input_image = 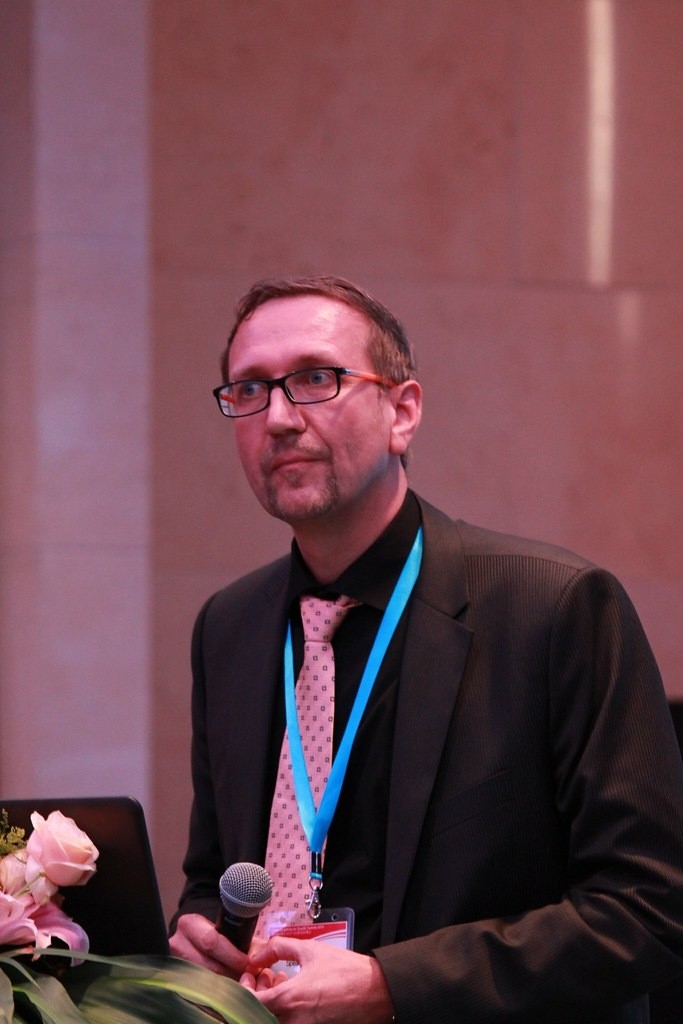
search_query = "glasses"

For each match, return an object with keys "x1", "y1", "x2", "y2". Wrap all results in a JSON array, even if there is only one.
[{"x1": 212, "y1": 365, "x2": 398, "y2": 418}]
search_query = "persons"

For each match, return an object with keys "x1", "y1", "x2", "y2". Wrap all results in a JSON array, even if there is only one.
[{"x1": 165, "y1": 275, "x2": 683, "y2": 1024}]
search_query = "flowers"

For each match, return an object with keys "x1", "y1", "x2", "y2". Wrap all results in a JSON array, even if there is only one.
[{"x1": 0, "y1": 807, "x2": 281, "y2": 1024}]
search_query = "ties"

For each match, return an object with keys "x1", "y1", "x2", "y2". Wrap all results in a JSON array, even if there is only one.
[{"x1": 252, "y1": 595, "x2": 363, "y2": 938}]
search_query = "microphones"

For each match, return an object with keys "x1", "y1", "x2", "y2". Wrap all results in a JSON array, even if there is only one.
[{"x1": 214, "y1": 862, "x2": 274, "y2": 955}]
